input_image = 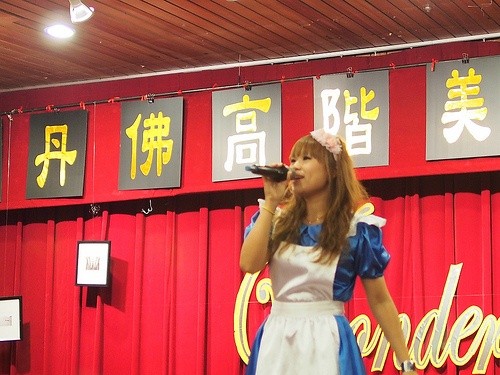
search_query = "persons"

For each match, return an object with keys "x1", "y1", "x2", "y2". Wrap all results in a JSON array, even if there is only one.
[{"x1": 238, "y1": 128, "x2": 416, "y2": 375}]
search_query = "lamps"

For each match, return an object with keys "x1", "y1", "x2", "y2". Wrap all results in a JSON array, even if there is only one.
[{"x1": 68, "y1": 0, "x2": 96, "y2": 25}]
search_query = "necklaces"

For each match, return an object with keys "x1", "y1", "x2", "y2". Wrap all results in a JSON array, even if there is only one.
[{"x1": 304, "y1": 212, "x2": 327, "y2": 225}]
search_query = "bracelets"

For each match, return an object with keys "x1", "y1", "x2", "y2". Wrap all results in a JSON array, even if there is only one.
[
  {"x1": 402, "y1": 360, "x2": 413, "y2": 372},
  {"x1": 263, "y1": 207, "x2": 275, "y2": 215}
]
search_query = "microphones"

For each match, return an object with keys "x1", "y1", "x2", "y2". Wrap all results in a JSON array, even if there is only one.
[{"x1": 245, "y1": 164, "x2": 296, "y2": 181}]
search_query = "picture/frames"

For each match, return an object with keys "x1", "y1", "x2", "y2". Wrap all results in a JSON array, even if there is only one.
[
  {"x1": 74, "y1": 240, "x2": 111, "y2": 286},
  {"x1": 0, "y1": 296, "x2": 23, "y2": 342}
]
